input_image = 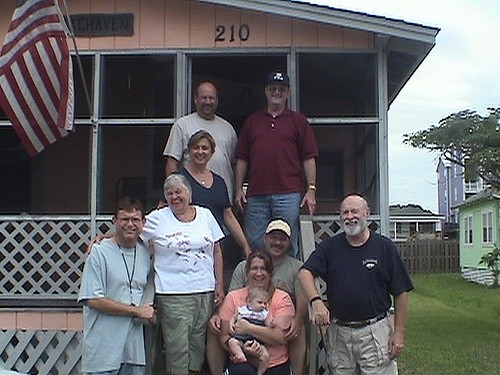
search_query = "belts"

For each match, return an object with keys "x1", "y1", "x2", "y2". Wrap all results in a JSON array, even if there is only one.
[{"x1": 329, "y1": 312, "x2": 388, "y2": 329}]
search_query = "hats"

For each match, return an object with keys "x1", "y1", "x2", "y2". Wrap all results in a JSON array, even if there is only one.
[
  {"x1": 265, "y1": 220, "x2": 292, "y2": 237},
  {"x1": 266, "y1": 70, "x2": 289, "y2": 86}
]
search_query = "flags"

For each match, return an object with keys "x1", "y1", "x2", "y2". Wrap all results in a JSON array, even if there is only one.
[{"x1": 0, "y1": 1, "x2": 74, "y2": 158}]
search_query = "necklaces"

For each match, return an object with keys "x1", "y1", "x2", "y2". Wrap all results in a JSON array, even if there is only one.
[
  {"x1": 190, "y1": 167, "x2": 208, "y2": 184},
  {"x1": 115, "y1": 234, "x2": 136, "y2": 307}
]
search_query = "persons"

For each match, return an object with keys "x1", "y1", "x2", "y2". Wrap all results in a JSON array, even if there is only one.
[
  {"x1": 87, "y1": 172, "x2": 227, "y2": 375},
  {"x1": 157, "y1": 130, "x2": 252, "y2": 258},
  {"x1": 218, "y1": 251, "x2": 295, "y2": 375},
  {"x1": 227, "y1": 288, "x2": 270, "y2": 375},
  {"x1": 78, "y1": 198, "x2": 155, "y2": 375},
  {"x1": 162, "y1": 82, "x2": 240, "y2": 257},
  {"x1": 207, "y1": 220, "x2": 307, "y2": 375},
  {"x1": 297, "y1": 195, "x2": 414, "y2": 375},
  {"x1": 235, "y1": 69, "x2": 319, "y2": 259}
]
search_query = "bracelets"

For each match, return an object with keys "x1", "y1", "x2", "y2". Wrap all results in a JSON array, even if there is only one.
[
  {"x1": 310, "y1": 297, "x2": 321, "y2": 307},
  {"x1": 307, "y1": 184, "x2": 316, "y2": 190}
]
